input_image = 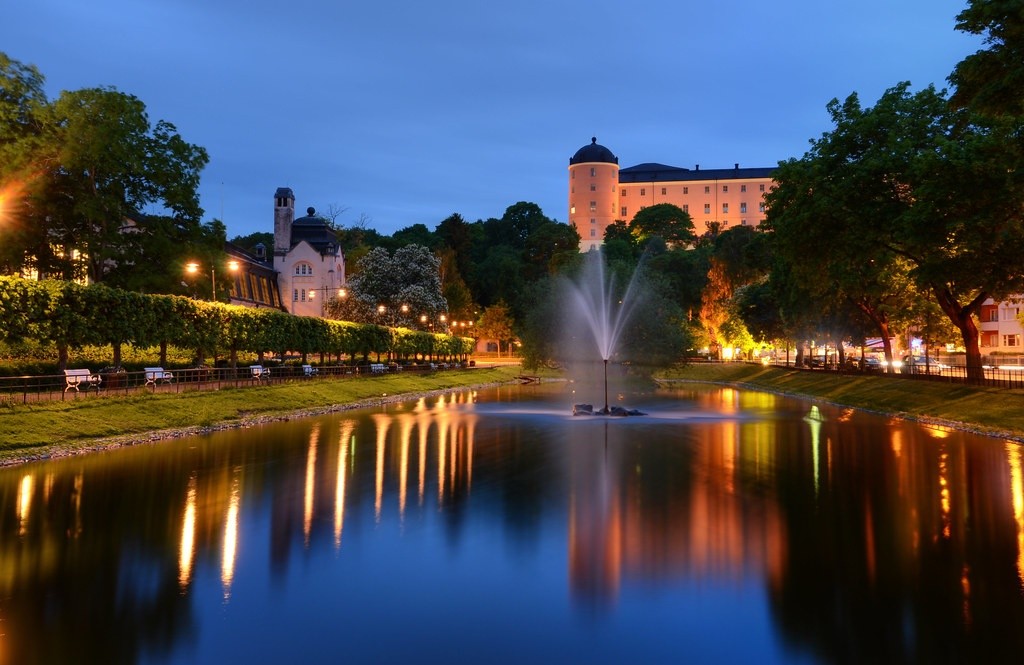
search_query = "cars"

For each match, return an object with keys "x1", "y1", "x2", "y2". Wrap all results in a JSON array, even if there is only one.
[
  {"x1": 852, "y1": 358, "x2": 860, "y2": 366},
  {"x1": 808, "y1": 355, "x2": 829, "y2": 367},
  {"x1": 864, "y1": 358, "x2": 881, "y2": 366}
]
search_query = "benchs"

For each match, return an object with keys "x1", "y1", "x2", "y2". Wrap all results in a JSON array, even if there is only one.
[
  {"x1": 249, "y1": 365, "x2": 270, "y2": 382},
  {"x1": 302, "y1": 365, "x2": 318, "y2": 377},
  {"x1": 369, "y1": 363, "x2": 462, "y2": 374},
  {"x1": 143, "y1": 367, "x2": 172, "y2": 386},
  {"x1": 63, "y1": 369, "x2": 102, "y2": 392}
]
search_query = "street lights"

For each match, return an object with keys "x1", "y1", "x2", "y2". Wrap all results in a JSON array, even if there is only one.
[
  {"x1": 185, "y1": 261, "x2": 240, "y2": 302},
  {"x1": 379, "y1": 305, "x2": 408, "y2": 328},
  {"x1": 421, "y1": 315, "x2": 445, "y2": 333},
  {"x1": 309, "y1": 288, "x2": 346, "y2": 320}
]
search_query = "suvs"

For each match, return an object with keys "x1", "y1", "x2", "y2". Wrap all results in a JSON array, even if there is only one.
[{"x1": 900, "y1": 355, "x2": 942, "y2": 375}]
